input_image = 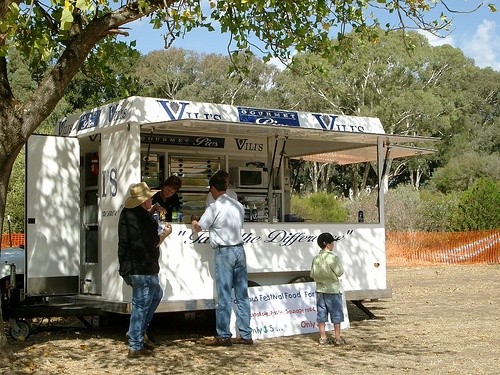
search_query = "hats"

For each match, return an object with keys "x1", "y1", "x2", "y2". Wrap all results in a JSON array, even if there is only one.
[
  {"x1": 318, "y1": 232, "x2": 340, "y2": 248},
  {"x1": 124, "y1": 182, "x2": 162, "y2": 208}
]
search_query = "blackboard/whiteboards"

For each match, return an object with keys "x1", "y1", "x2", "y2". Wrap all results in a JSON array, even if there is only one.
[{"x1": 229, "y1": 279, "x2": 351, "y2": 339}]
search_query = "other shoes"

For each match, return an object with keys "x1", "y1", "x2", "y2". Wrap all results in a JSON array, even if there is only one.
[
  {"x1": 129, "y1": 346, "x2": 150, "y2": 357},
  {"x1": 143, "y1": 344, "x2": 154, "y2": 350},
  {"x1": 336, "y1": 338, "x2": 346, "y2": 345},
  {"x1": 320, "y1": 338, "x2": 329, "y2": 345},
  {"x1": 234, "y1": 338, "x2": 252, "y2": 344},
  {"x1": 206, "y1": 337, "x2": 231, "y2": 346}
]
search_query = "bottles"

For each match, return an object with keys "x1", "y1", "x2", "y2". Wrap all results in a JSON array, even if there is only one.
[
  {"x1": 171, "y1": 207, "x2": 183, "y2": 223},
  {"x1": 243, "y1": 204, "x2": 258, "y2": 222},
  {"x1": 153, "y1": 210, "x2": 159, "y2": 222},
  {"x1": 358, "y1": 209, "x2": 363, "y2": 223}
]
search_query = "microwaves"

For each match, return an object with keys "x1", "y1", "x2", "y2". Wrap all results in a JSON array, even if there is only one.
[{"x1": 230, "y1": 165, "x2": 269, "y2": 189}]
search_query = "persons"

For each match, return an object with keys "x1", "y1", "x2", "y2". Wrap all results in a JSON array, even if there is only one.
[
  {"x1": 151, "y1": 176, "x2": 183, "y2": 223},
  {"x1": 117, "y1": 182, "x2": 172, "y2": 357},
  {"x1": 309, "y1": 233, "x2": 346, "y2": 346},
  {"x1": 191, "y1": 170, "x2": 253, "y2": 346}
]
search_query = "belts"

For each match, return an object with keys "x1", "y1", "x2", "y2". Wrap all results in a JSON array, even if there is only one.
[{"x1": 217, "y1": 243, "x2": 243, "y2": 248}]
